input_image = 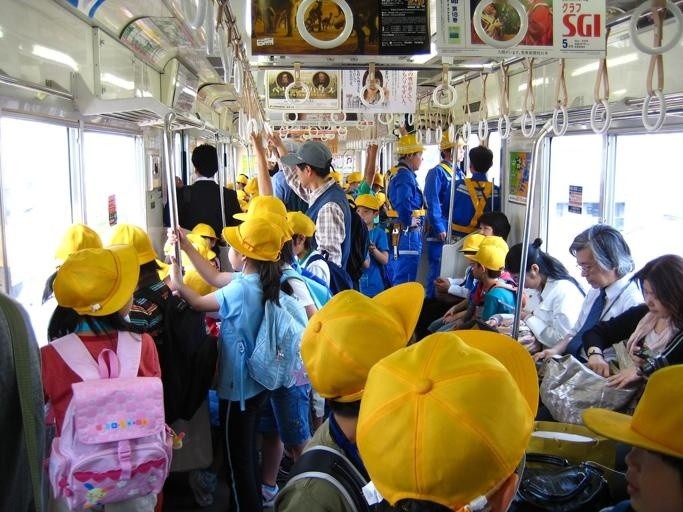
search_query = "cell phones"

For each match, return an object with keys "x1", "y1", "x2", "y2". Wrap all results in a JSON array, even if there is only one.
[{"x1": 498, "y1": 300, "x2": 517, "y2": 310}]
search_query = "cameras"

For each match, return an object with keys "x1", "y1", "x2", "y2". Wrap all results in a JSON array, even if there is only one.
[{"x1": 631, "y1": 332, "x2": 682, "y2": 373}]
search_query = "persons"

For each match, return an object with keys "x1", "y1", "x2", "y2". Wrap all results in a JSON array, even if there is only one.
[
  {"x1": 160, "y1": 224, "x2": 221, "y2": 296},
  {"x1": 287, "y1": 211, "x2": 330, "y2": 288},
  {"x1": 265, "y1": 130, "x2": 291, "y2": 204},
  {"x1": 485, "y1": 240, "x2": 587, "y2": 355},
  {"x1": 225, "y1": 174, "x2": 258, "y2": 211},
  {"x1": 443, "y1": 146, "x2": 500, "y2": 240},
  {"x1": 582, "y1": 255, "x2": 683, "y2": 388},
  {"x1": 268, "y1": 131, "x2": 352, "y2": 271},
  {"x1": 580, "y1": 364, "x2": 682, "y2": 512},
  {"x1": 163, "y1": 144, "x2": 243, "y2": 245},
  {"x1": 110, "y1": 223, "x2": 171, "y2": 368},
  {"x1": 329, "y1": 143, "x2": 390, "y2": 210},
  {"x1": 354, "y1": 194, "x2": 389, "y2": 298},
  {"x1": 38, "y1": 244, "x2": 187, "y2": 511},
  {"x1": 0, "y1": 293, "x2": 51, "y2": 512},
  {"x1": 428, "y1": 210, "x2": 517, "y2": 331},
  {"x1": 232, "y1": 197, "x2": 319, "y2": 505},
  {"x1": 47, "y1": 223, "x2": 102, "y2": 262},
  {"x1": 423, "y1": 131, "x2": 468, "y2": 299},
  {"x1": 385, "y1": 125, "x2": 425, "y2": 286},
  {"x1": 530, "y1": 224, "x2": 643, "y2": 362},
  {"x1": 362, "y1": 67, "x2": 387, "y2": 109},
  {"x1": 169, "y1": 219, "x2": 284, "y2": 512},
  {"x1": 272, "y1": 282, "x2": 424, "y2": 511}
]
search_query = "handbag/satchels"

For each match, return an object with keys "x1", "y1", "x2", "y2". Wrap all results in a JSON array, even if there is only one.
[
  {"x1": 507, "y1": 452, "x2": 628, "y2": 512},
  {"x1": 522, "y1": 420, "x2": 618, "y2": 471},
  {"x1": 538, "y1": 339, "x2": 642, "y2": 427}
]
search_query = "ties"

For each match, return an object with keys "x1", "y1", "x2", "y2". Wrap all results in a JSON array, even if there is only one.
[{"x1": 562, "y1": 287, "x2": 607, "y2": 357}]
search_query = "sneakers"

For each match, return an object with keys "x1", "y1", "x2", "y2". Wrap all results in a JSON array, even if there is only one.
[
  {"x1": 279, "y1": 454, "x2": 294, "y2": 476},
  {"x1": 258, "y1": 483, "x2": 280, "y2": 508}
]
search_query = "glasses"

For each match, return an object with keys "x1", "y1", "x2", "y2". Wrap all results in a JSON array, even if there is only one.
[{"x1": 575, "y1": 264, "x2": 595, "y2": 272}]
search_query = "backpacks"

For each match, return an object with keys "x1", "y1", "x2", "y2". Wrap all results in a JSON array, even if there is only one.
[
  {"x1": 289, "y1": 188, "x2": 308, "y2": 213},
  {"x1": 278, "y1": 261, "x2": 334, "y2": 305},
  {"x1": 133, "y1": 287, "x2": 218, "y2": 421},
  {"x1": 47, "y1": 330, "x2": 186, "y2": 510},
  {"x1": 245, "y1": 290, "x2": 310, "y2": 391},
  {"x1": 306, "y1": 250, "x2": 354, "y2": 294},
  {"x1": 347, "y1": 199, "x2": 370, "y2": 279}
]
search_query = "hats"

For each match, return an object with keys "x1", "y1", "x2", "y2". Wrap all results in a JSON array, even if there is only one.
[
  {"x1": 342, "y1": 183, "x2": 351, "y2": 189},
  {"x1": 191, "y1": 222, "x2": 219, "y2": 239},
  {"x1": 288, "y1": 212, "x2": 318, "y2": 238},
  {"x1": 374, "y1": 173, "x2": 384, "y2": 187},
  {"x1": 280, "y1": 140, "x2": 333, "y2": 168},
  {"x1": 463, "y1": 244, "x2": 507, "y2": 271},
  {"x1": 108, "y1": 224, "x2": 159, "y2": 266},
  {"x1": 221, "y1": 216, "x2": 286, "y2": 262},
  {"x1": 252, "y1": 211, "x2": 294, "y2": 242},
  {"x1": 376, "y1": 192, "x2": 386, "y2": 207},
  {"x1": 248, "y1": 193, "x2": 259, "y2": 205},
  {"x1": 459, "y1": 234, "x2": 486, "y2": 252},
  {"x1": 55, "y1": 224, "x2": 103, "y2": 262},
  {"x1": 354, "y1": 194, "x2": 380, "y2": 211},
  {"x1": 479, "y1": 235, "x2": 509, "y2": 255},
  {"x1": 237, "y1": 200, "x2": 248, "y2": 210},
  {"x1": 329, "y1": 170, "x2": 342, "y2": 182},
  {"x1": 243, "y1": 176, "x2": 258, "y2": 194},
  {"x1": 345, "y1": 192, "x2": 356, "y2": 208},
  {"x1": 182, "y1": 270, "x2": 217, "y2": 295},
  {"x1": 232, "y1": 195, "x2": 287, "y2": 221},
  {"x1": 395, "y1": 133, "x2": 426, "y2": 155},
  {"x1": 439, "y1": 128, "x2": 467, "y2": 149},
  {"x1": 154, "y1": 257, "x2": 171, "y2": 281},
  {"x1": 181, "y1": 240, "x2": 207, "y2": 267},
  {"x1": 581, "y1": 363, "x2": 683, "y2": 459},
  {"x1": 299, "y1": 281, "x2": 426, "y2": 404},
  {"x1": 184, "y1": 233, "x2": 217, "y2": 260},
  {"x1": 226, "y1": 182, "x2": 234, "y2": 189},
  {"x1": 347, "y1": 172, "x2": 364, "y2": 183},
  {"x1": 236, "y1": 174, "x2": 248, "y2": 185},
  {"x1": 52, "y1": 243, "x2": 141, "y2": 317},
  {"x1": 236, "y1": 190, "x2": 246, "y2": 199}
]
